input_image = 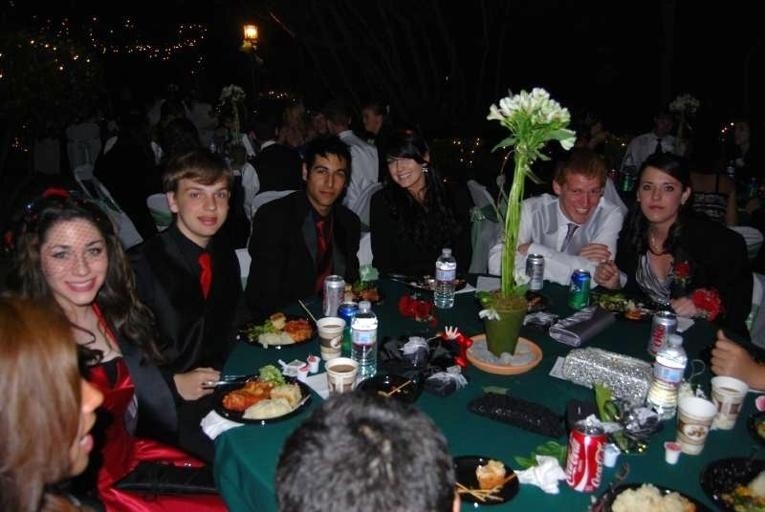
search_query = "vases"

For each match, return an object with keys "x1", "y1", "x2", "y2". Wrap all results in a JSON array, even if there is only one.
[{"x1": 481, "y1": 291, "x2": 529, "y2": 358}]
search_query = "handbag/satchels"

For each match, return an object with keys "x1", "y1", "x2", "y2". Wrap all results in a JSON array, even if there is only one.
[
  {"x1": 548, "y1": 303, "x2": 617, "y2": 347},
  {"x1": 467, "y1": 391, "x2": 564, "y2": 438}
]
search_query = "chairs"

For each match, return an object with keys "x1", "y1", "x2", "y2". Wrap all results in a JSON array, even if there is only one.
[
  {"x1": 347, "y1": 182, "x2": 383, "y2": 230},
  {"x1": 74, "y1": 161, "x2": 99, "y2": 203},
  {"x1": 467, "y1": 178, "x2": 501, "y2": 225},
  {"x1": 90, "y1": 180, "x2": 142, "y2": 255},
  {"x1": 746, "y1": 274, "x2": 764, "y2": 335},
  {"x1": 246, "y1": 190, "x2": 295, "y2": 219},
  {"x1": 357, "y1": 232, "x2": 373, "y2": 269},
  {"x1": 728, "y1": 225, "x2": 764, "y2": 260},
  {"x1": 147, "y1": 194, "x2": 174, "y2": 232},
  {"x1": 235, "y1": 248, "x2": 252, "y2": 290}
]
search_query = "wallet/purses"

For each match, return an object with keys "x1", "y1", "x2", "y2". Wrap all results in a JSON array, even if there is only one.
[{"x1": 559, "y1": 346, "x2": 656, "y2": 408}]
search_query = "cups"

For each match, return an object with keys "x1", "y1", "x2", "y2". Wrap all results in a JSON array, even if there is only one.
[
  {"x1": 317, "y1": 316, "x2": 346, "y2": 362},
  {"x1": 710, "y1": 376, "x2": 748, "y2": 430},
  {"x1": 325, "y1": 357, "x2": 358, "y2": 391},
  {"x1": 677, "y1": 397, "x2": 716, "y2": 456}
]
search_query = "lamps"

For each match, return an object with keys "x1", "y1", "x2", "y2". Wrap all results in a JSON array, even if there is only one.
[{"x1": 241, "y1": 21, "x2": 261, "y2": 47}]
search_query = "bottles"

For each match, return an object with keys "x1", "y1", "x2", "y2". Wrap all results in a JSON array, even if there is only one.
[
  {"x1": 644, "y1": 331, "x2": 688, "y2": 422},
  {"x1": 338, "y1": 301, "x2": 358, "y2": 359},
  {"x1": 434, "y1": 248, "x2": 455, "y2": 310},
  {"x1": 567, "y1": 268, "x2": 591, "y2": 311},
  {"x1": 525, "y1": 252, "x2": 545, "y2": 291},
  {"x1": 647, "y1": 310, "x2": 679, "y2": 357},
  {"x1": 322, "y1": 273, "x2": 346, "y2": 319},
  {"x1": 349, "y1": 299, "x2": 378, "y2": 380},
  {"x1": 564, "y1": 422, "x2": 607, "y2": 495}
]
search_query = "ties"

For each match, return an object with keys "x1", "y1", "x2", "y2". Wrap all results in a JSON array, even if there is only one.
[
  {"x1": 314, "y1": 219, "x2": 333, "y2": 293},
  {"x1": 561, "y1": 223, "x2": 579, "y2": 254},
  {"x1": 196, "y1": 251, "x2": 213, "y2": 300}
]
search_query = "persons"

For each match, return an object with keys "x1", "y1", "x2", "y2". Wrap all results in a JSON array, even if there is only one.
[
  {"x1": 726, "y1": 119, "x2": 765, "y2": 228},
  {"x1": 63, "y1": 103, "x2": 102, "y2": 175},
  {"x1": 713, "y1": 329, "x2": 765, "y2": 392},
  {"x1": 17, "y1": 189, "x2": 226, "y2": 512},
  {"x1": 0, "y1": 293, "x2": 105, "y2": 511},
  {"x1": 324, "y1": 99, "x2": 378, "y2": 238},
  {"x1": 148, "y1": 80, "x2": 246, "y2": 150},
  {"x1": 241, "y1": 115, "x2": 304, "y2": 222},
  {"x1": 250, "y1": 107, "x2": 277, "y2": 133},
  {"x1": 309, "y1": 108, "x2": 330, "y2": 135},
  {"x1": 369, "y1": 130, "x2": 473, "y2": 284},
  {"x1": 488, "y1": 150, "x2": 623, "y2": 288},
  {"x1": 593, "y1": 152, "x2": 753, "y2": 353},
  {"x1": 279, "y1": 96, "x2": 315, "y2": 151},
  {"x1": 244, "y1": 133, "x2": 362, "y2": 326},
  {"x1": 619, "y1": 112, "x2": 688, "y2": 187},
  {"x1": 273, "y1": 390, "x2": 464, "y2": 512},
  {"x1": 94, "y1": 112, "x2": 165, "y2": 254},
  {"x1": 541, "y1": 112, "x2": 618, "y2": 153},
  {"x1": 121, "y1": 150, "x2": 244, "y2": 458},
  {"x1": 362, "y1": 99, "x2": 412, "y2": 140}
]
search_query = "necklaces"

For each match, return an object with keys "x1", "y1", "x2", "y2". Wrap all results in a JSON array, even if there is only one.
[{"x1": 645, "y1": 230, "x2": 672, "y2": 257}]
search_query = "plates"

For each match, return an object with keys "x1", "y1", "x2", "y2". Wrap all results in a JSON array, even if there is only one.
[
  {"x1": 410, "y1": 275, "x2": 468, "y2": 292},
  {"x1": 591, "y1": 484, "x2": 708, "y2": 512},
  {"x1": 210, "y1": 373, "x2": 313, "y2": 424},
  {"x1": 747, "y1": 411, "x2": 765, "y2": 447},
  {"x1": 699, "y1": 456, "x2": 765, "y2": 512},
  {"x1": 466, "y1": 334, "x2": 542, "y2": 376},
  {"x1": 452, "y1": 455, "x2": 519, "y2": 505},
  {"x1": 240, "y1": 315, "x2": 316, "y2": 350}
]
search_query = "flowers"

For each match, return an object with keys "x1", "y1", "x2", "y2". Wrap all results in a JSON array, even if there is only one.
[
  {"x1": 219, "y1": 85, "x2": 246, "y2": 145},
  {"x1": 477, "y1": 87, "x2": 576, "y2": 303}
]
